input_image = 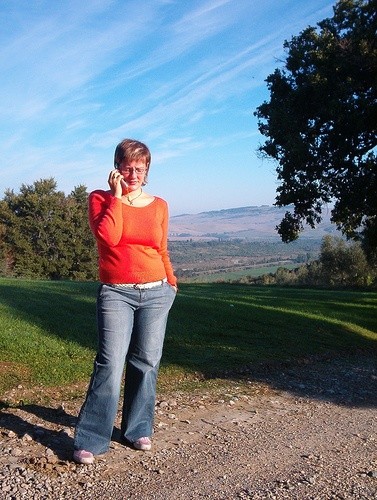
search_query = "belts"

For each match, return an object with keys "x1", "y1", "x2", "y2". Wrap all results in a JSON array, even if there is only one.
[{"x1": 102, "y1": 278, "x2": 168, "y2": 291}]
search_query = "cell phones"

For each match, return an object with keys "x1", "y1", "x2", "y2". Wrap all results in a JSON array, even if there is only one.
[{"x1": 116, "y1": 173, "x2": 129, "y2": 195}]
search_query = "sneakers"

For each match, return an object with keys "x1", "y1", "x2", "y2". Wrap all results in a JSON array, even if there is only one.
[
  {"x1": 74, "y1": 445, "x2": 95, "y2": 463},
  {"x1": 133, "y1": 436, "x2": 153, "y2": 451}
]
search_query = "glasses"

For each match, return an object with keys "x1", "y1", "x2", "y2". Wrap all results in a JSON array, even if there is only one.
[{"x1": 117, "y1": 167, "x2": 148, "y2": 173}]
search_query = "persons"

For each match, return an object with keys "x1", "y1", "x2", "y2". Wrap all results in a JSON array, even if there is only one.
[{"x1": 72, "y1": 138, "x2": 179, "y2": 465}]
